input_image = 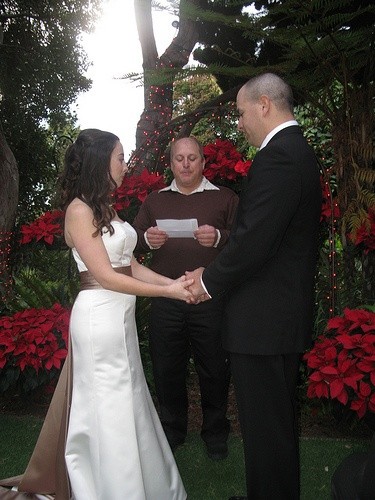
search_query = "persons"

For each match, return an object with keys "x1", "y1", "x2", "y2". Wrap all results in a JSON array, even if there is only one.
[
  {"x1": 185, "y1": 73, "x2": 323, "y2": 500},
  {"x1": 132, "y1": 136, "x2": 241, "y2": 461},
  {"x1": 0, "y1": 129, "x2": 195, "y2": 500}
]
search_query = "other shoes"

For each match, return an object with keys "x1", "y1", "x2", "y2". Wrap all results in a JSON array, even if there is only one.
[
  {"x1": 166, "y1": 431, "x2": 186, "y2": 447},
  {"x1": 206, "y1": 442, "x2": 229, "y2": 460}
]
